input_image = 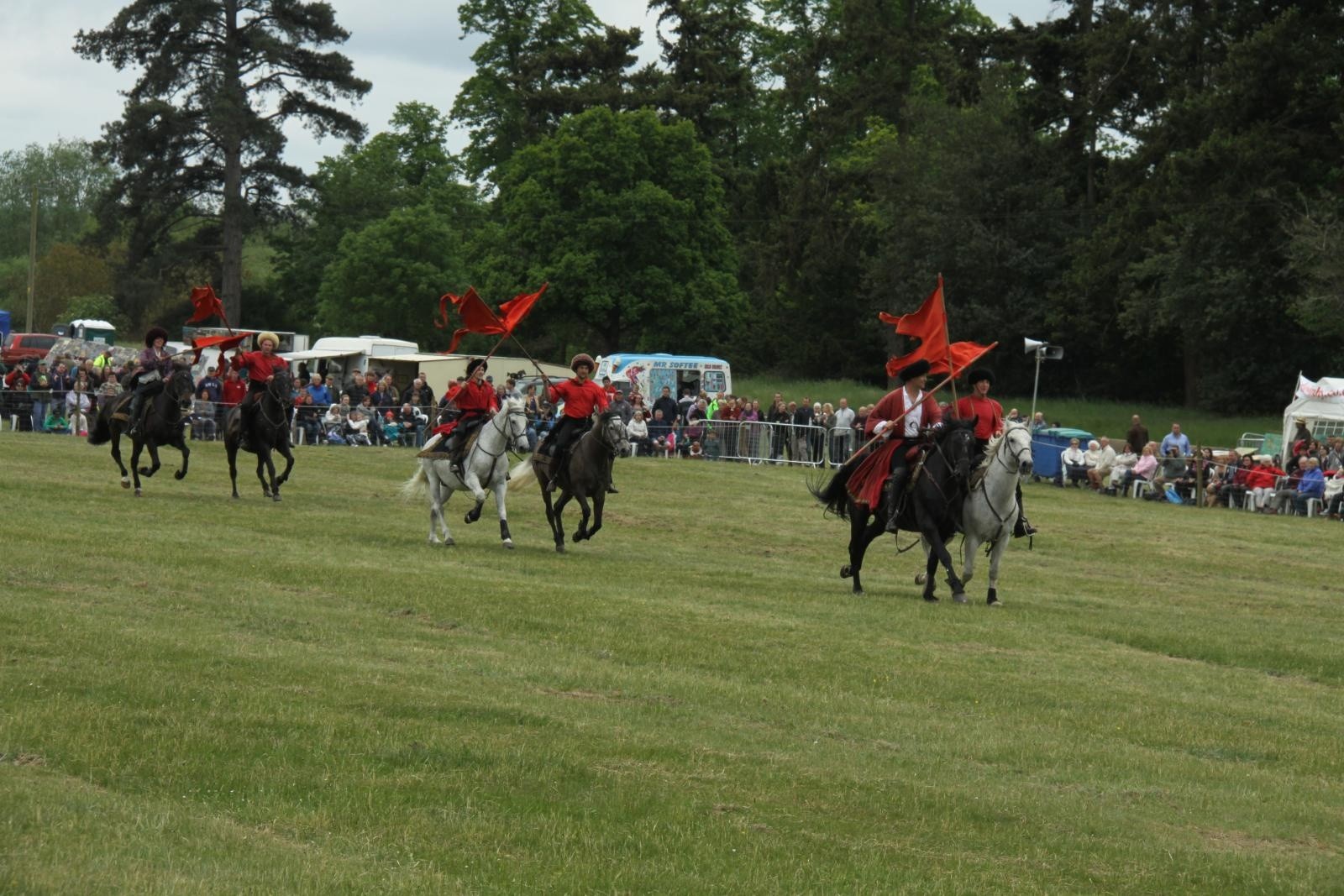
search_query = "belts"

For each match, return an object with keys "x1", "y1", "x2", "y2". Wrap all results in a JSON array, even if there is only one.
[
  {"x1": 897, "y1": 436, "x2": 918, "y2": 442},
  {"x1": 468, "y1": 409, "x2": 486, "y2": 413}
]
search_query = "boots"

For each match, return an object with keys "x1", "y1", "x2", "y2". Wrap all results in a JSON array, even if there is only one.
[
  {"x1": 1014, "y1": 482, "x2": 1037, "y2": 538},
  {"x1": 885, "y1": 482, "x2": 903, "y2": 533}
]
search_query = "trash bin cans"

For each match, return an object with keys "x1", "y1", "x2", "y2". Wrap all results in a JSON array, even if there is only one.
[{"x1": 1029, "y1": 428, "x2": 1093, "y2": 485}]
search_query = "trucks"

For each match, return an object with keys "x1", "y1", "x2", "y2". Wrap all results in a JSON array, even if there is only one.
[{"x1": 164, "y1": 327, "x2": 310, "y2": 388}]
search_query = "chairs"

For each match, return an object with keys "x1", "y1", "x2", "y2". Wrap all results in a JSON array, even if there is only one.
[
  {"x1": 0, "y1": 363, "x2": 37, "y2": 433},
  {"x1": 1061, "y1": 449, "x2": 1325, "y2": 518}
]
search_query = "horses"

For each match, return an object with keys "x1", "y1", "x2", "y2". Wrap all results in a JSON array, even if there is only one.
[
  {"x1": 402, "y1": 396, "x2": 530, "y2": 550},
  {"x1": 506, "y1": 405, "x2": 632, "y2": 554},
  {"x1": 804, "y1": 408, "x2": 1033, "y2": 607},
  {"x1": 224, "y1": 363, "x2": 294, "y2": 502},
  {"x1": 88, "y1": 362, "x2": 196, "y2": 497}
]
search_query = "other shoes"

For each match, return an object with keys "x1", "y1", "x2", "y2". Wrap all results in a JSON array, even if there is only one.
[
  {"x1": 240, "y1": 438, "x2": 250, "y2": 451},
  {"x1": 129, "y1": 427, "x2": 139, "y2": 436},
  {"x1": 1265, "y1": 508, "x2": 1278, "y2": 514},
  {"x1": 505, "y1": 474, "x2": 511, "y2": 481},
  {"x1": 449, "y1": 462, "x2": 459, "y2": 472},
  {"x1": 606, "y1": 484, "x2": 619, "y2": 493},
  {"x1": 545, "y1": 481, "x2": 557, "y2": 492}
]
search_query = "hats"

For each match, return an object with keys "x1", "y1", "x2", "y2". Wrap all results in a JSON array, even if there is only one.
[
  {"x1": 39, "y1": 359, "x2": 47, "y2": 366},
  {"x1": 145, "y1": 328, "x2": 168, "y2": 348},
  {"x1": 385, "y1": 411, "x2": 394, "y2": 418},
  {"x1": 52, "y1": 404, "x2": 62, "y2": 414},
  {"x1": 1291, "y1": 417, "x2": 1308, "y2": 423},
  {"x1": 899, "y1": 359, "x2": 930, "y2": 380},
  {"x1": 616, "y1": 390, "x2": 623, "y2": 395},
  {"x1": 486, "y1": 375, "x2": 493, "y2": 382},
  {"x1": 256, "y1": 332, "x2": 279, "y2": 350},
  {"x1": 466, "y1": 357, "x2": 487, "y2": 375},
  {"x1": 351, "y1": 367, "x2": 360, "y2": 373},
  {"x1": 570, "y1": 353, "x2": 594, "y2": 373},
  {"x1": 326, "y1": 374, "x2": 334, "y2": 381},
  {"x1": 207, "y1": 366, "x2": 214, "y2": 373},
  {"x1": 968, "y1": 366, "x2": 995, "y2": 386}
]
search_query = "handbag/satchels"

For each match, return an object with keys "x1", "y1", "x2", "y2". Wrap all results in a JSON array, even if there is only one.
[{"x1": 1164, "y1": 488, "x2": 1183, "y2": 504}]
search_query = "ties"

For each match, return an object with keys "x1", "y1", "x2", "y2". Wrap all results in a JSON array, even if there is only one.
[{"x1": 1322, "y1": 458, "x2": 1328, "y2": 472}]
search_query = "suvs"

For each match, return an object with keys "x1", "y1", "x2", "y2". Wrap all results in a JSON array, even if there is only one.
[
  {"x1": 514, "y1": 375, "x2": 574, "y2": 407},
  {"x1": 1, "y1": 333, "x2": 63, "y2": 373}
]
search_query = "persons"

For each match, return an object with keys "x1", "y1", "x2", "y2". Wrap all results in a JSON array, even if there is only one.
[
  {"x1": 127, "y1": 326, "x2": 177, "y2": 438},
  {"x1": 446, "y1": 359, "x2": 511, "y2": 481},
  {"x1": 601, "y1": 375, "x2": 950, "y2": 470},
  {"x1": 190, "y1": 365, "x2": 246, "y2": 442},
  {"x1": 4, "y1": 347, "x2": 141, "y2": 435},
  {"x1": 944, "y1": 367, "x2": 1038, "y2": 540},
  {"x1": 291, "y1": 368, "x2": 565, "y2": 453},
  {"x1": 1007, "y1": 408, "x2": 1061, "y2": 432},
  {"x1": 865, "y1": 359, "x2": 943, "y2": 534},
  {"x1": 540, "y1": 353, "x2": 619, "y2": 494},
  {"x1": 231, "y1": 331, "x2": 295, "y2": 449},
  {"x1": 1063, "y1": 409, "x2": 1344, "y2": 522}
]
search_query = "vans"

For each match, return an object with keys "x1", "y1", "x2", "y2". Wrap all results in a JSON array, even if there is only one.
[{"x1": 594, "y1": 353, "x2": 732, "y2": 422}]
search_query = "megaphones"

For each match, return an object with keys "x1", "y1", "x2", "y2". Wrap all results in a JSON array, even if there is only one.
[
  {"x1": 1041, "y1": 347, "x2": 1063, "y2": 359},
  {"x1": 1024, "y1": 337, "x2": 1049, "y2": 354}
]
